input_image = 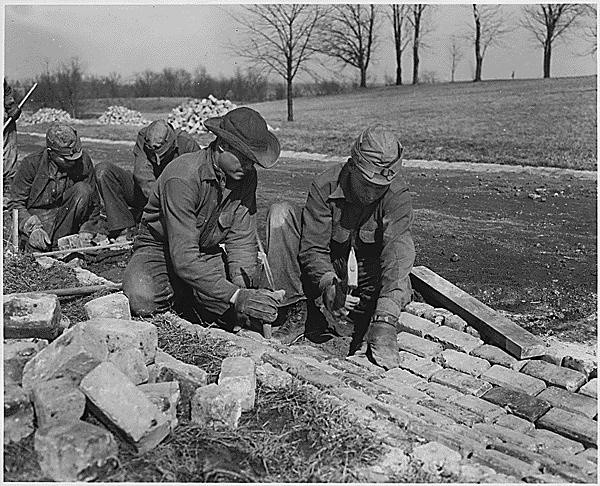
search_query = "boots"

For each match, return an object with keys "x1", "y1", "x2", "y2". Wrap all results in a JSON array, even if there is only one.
[{"x1": 268, "y1": 310, "x2": 324, "y2": 347}]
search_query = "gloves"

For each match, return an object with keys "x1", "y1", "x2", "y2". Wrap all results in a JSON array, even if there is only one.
[
  {"x1": 234, "y1": 288, "x2": 285, "y2": 323},
  {"x1": 317, "y1": 272, "x2": 361, "y2": 319},
  {"x1": 366, "y1": 314, "x2": 402, "y2": 369},
  {"x1": 24, "y1": 215, "x2": 51, "y2": 251}
]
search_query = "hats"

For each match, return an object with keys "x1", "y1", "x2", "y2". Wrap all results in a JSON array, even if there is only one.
[
  {"x1": 46, "y1": 123, "x2": 83, "y2": 161},
  {"x1": 203, "y1": 107, "x2": 281, "y2": 169},
  {"x1": 352, "y1": 123, "x2": 403, "y2": 186},
  {"x1": 144, "y1": 119, "x2": 177, "y2": 160}
]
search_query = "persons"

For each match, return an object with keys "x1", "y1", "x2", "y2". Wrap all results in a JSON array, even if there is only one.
[
  {"x1": 8, "y1": 124, "x2": 102, "y2": 256},
  {"x1": 94, "y1": 118, "x2": 201, "y2": 244},
  {"x1": 3, "y1": 77, "x2": 21, "y2": 210},
  {"x1": 256, "y1": 123, "x2": 416, "y2": 370},
  {"x1": 118, "y1": 105, "x2": 283, "y2": 324}
]
